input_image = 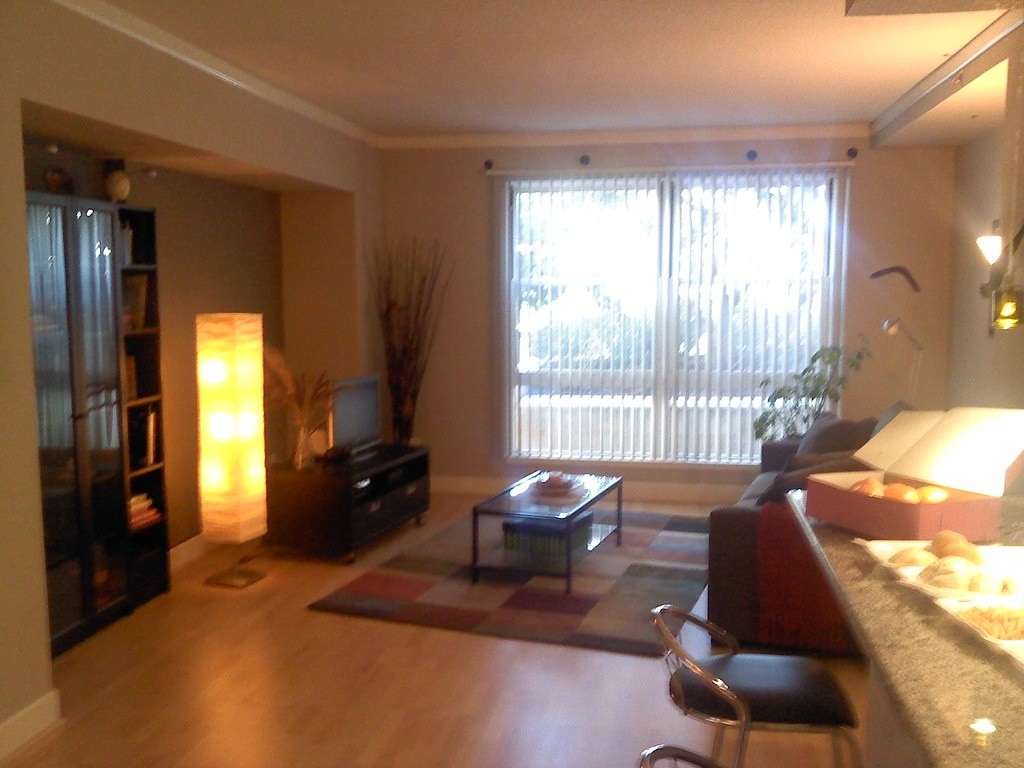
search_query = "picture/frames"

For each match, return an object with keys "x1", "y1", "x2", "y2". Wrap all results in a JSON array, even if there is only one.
[{"x1": 123, "y1": 276, "x2": 148, "y2": 328}]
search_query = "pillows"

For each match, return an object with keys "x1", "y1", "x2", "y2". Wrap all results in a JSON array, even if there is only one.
[
  {"x1": 779, "y1": 451, "x2": 860, "y2": 474},
  {"x1": 796, "y1": 412, "x2": 877, "y2": 458},
  {"x1": 760, "y1": 459, "x2": 871, "y2": 503}
]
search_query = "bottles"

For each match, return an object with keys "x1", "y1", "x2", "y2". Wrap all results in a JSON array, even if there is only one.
[{"x1": 43, "y1": 143, "x2": 81, "y2": 198}]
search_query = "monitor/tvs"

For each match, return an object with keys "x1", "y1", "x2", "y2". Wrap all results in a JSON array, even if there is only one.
[{"x1": 329, "y1": 375, "x2": 383, "y2": 462}]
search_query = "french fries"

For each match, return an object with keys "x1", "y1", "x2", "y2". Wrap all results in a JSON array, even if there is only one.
[{"x1": 954, "y1": 604, "x2": 1023, "y2": 641}]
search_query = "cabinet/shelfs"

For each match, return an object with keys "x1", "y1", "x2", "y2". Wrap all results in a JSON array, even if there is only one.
[
  {"x1": 23, "y1": 192, "x2": 170, "y2": 658},
  {"x1": 268, "y1": 442, "x2": 429, "y2": 564}
]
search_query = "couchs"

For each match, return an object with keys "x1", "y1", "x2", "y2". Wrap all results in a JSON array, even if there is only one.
[{"x1": 708, "y1": 400, "x2": 915, "y2": 651}]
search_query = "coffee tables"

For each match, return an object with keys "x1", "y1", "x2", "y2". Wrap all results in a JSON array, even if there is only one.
[{"x1": 472, "y1": 470, "x2": 621, "y2": 588}]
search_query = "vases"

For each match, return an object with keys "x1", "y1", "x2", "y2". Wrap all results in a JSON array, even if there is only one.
[{"x1": 294, "y1": 427, "x2": 315, "y2": 472}]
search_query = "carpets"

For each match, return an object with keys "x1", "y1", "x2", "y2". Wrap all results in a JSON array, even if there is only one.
[{"x1": 308, "y1": 507, "x2": 710, "y2": 658}]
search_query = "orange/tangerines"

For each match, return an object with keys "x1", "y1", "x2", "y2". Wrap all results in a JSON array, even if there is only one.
[{"x1": 847, "y1": 480, "x2": 951, "y2": 503}]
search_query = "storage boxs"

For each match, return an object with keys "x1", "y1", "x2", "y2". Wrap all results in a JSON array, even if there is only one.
[
  {"x1": 503, "y1": 511, "x2": 593, "y2": 556},
  {"x1": 805, "y1": 403, "x2": 1024, "y2": 540}
]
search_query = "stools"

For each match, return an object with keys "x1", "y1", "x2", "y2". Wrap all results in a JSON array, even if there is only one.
[{"x1": 638, "y1": 603, "x2": 865, "y2": 768}]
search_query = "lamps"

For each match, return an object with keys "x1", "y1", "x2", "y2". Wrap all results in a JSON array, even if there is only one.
[
  {"x1": 883, "y1": 316, "x2": 923, "y2": 400},
  {"x1": 991, "y1": 146, "x2": 1024, "y2": 330},
  {"x1": 196, "y1": 314, "x2": 267, "y2": 587}
]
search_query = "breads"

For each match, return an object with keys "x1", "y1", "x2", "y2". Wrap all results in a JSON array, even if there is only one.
[{"x1": 891, "y1": 528, "x2": 1016, "y2": 596}]
